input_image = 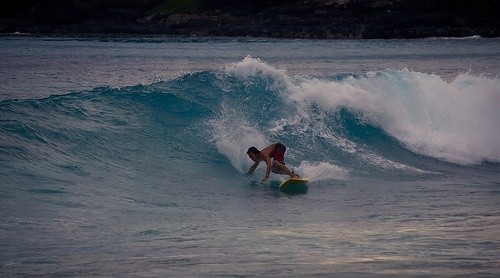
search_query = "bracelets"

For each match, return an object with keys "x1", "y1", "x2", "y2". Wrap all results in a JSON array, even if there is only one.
[{"x1": 266, "y1": 176, "x2": 270, "y2": 178}]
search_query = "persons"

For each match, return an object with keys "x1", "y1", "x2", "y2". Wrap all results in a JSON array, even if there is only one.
[{"x1": 247, "y1": 142, "x2": 300, "y2": 183}]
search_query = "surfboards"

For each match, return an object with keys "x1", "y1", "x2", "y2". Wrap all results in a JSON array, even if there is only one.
[{"x1": 279, "y1": 177, "x2": 309, "y2": 189}]
